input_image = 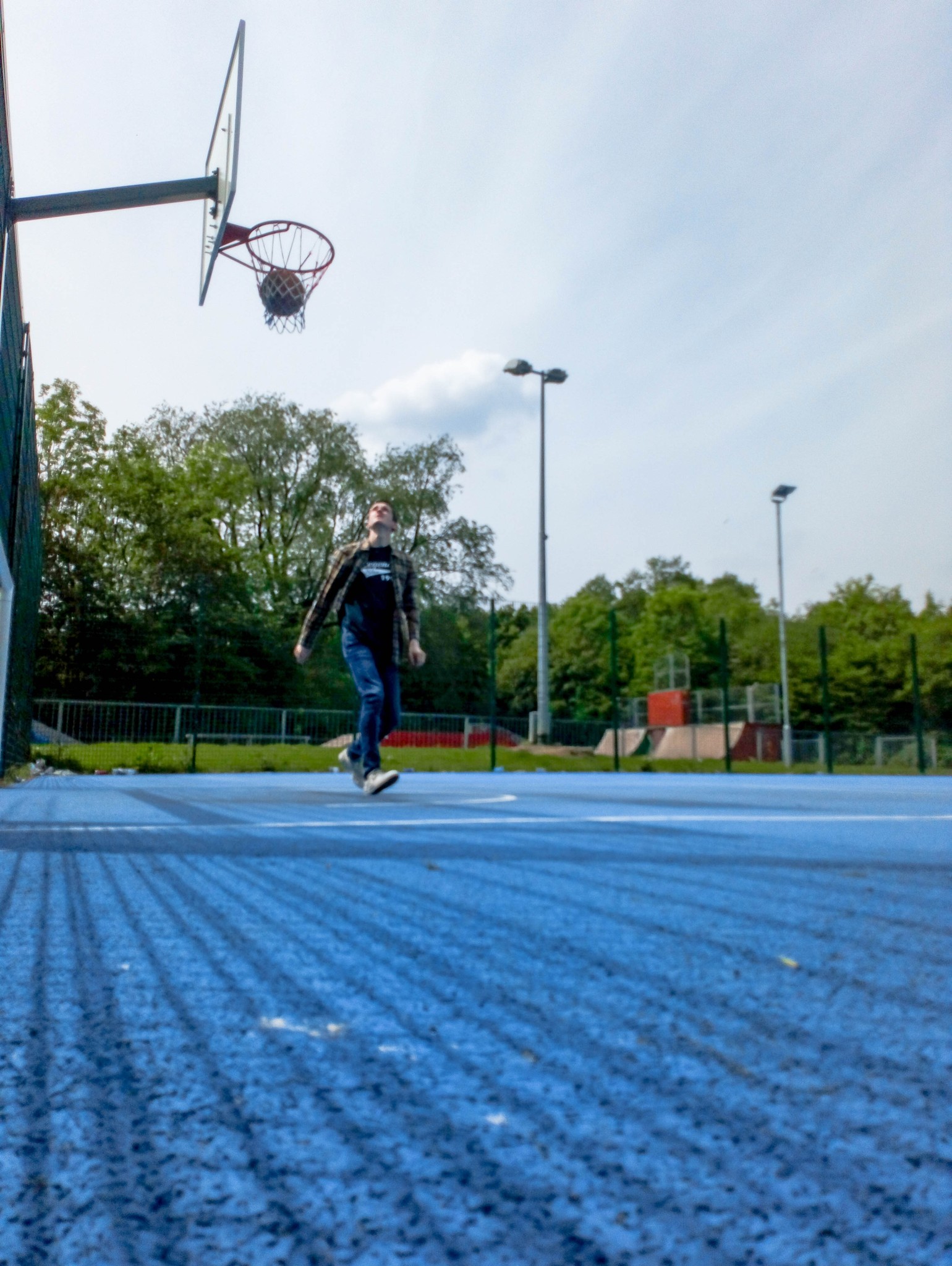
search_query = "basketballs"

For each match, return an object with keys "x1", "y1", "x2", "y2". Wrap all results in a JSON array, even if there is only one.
[{"x1": 259, "y1": 268, "x2": 306, "y2": 317}]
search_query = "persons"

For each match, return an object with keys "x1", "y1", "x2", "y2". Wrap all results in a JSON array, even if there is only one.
[{"x1": 294, "y1": 498, "x2": 426, "y2": 796}]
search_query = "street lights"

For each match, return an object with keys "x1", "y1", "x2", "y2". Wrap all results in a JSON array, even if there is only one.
[
  {"x1": 769, "y1": 483, "x2": 798, "y2": 768},
  {"x1": 502, "y1": 358, "x2": 568, "y2": 746}
]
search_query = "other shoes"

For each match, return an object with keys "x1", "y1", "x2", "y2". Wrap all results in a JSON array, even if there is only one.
[
  {"x1": 363, "y1": 769, "x2": 399, "y2": 797},
  {"x1": 337, "y1": 748, "x2": 365, "y2": 789}
]
